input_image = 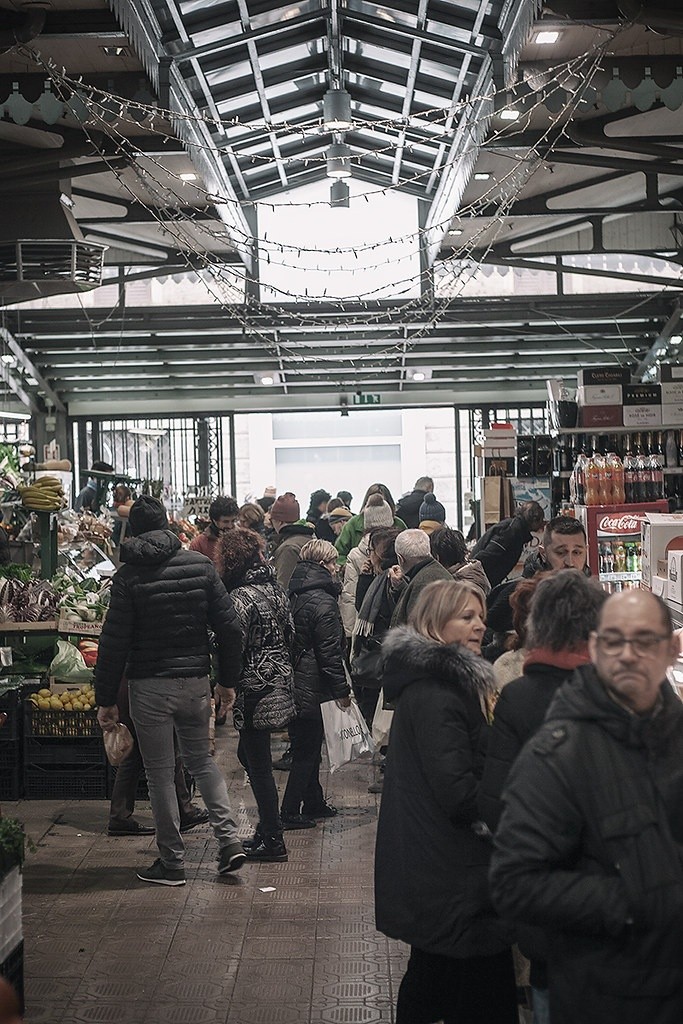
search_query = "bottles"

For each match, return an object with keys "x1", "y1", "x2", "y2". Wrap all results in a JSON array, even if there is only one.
[
  {"x1": 569, "y1": 453, "x2": 665, "y2": 505},
  {"x1": 555, "y1": 479, "x2": 569, "y2": 515},
  {"x1": 599, "y1": 540, "x2": 644, "y2": 574},
  {"x1": 666, "y1": 475, "x2": 683, "y2": 508},
  {"x1": 554, "y1": 429, "x2": 683, "y2": 470}
]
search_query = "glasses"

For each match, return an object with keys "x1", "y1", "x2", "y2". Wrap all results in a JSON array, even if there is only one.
[
  {"x1": 366, "y1": 548, "x2": 375, "y2": 556},
  {"x1": 596, "y1": 633, "x2": 666, "y2": 658}
]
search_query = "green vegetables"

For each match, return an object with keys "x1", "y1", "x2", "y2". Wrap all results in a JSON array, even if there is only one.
[
  {"x1": 0, "y1": 815, "x2": 38, "y2": 856},
  {"x1": 0, "y1": 560, "x2": 34, "y2": 582}
]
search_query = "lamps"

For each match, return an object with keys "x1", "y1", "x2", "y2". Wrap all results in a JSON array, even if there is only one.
[
  {"x1": 326, "y1": 133, "x2": 352, "y2": 177},
  {"x1": 323, "y1": 69, "x2": 353, "y2": 131},
  {"x1": 329, "y1": 178, "x2": 350, "y2": 209}
]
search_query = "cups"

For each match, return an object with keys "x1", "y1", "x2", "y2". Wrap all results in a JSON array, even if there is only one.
[{"x1": 549, "y1": 400, "x2": 577, "y2": 428}]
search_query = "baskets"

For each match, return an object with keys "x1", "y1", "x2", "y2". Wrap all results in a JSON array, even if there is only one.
[{"x1": 0, "y1": 693, "x2": 150, "y2": 801}]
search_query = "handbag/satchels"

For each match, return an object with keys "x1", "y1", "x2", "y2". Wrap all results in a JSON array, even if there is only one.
[
  {"x1": 371, "y1": 686, "x2": 395, "y2": 748},
  {"x1": 320, "y1": 697, "x2": 375, "y2": 773}
]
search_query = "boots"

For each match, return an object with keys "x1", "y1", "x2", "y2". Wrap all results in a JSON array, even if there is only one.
[{"x1": 243, "y1": 824, "x2": 289, "y2": 861}]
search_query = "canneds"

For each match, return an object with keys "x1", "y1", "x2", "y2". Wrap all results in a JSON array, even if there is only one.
[{"x1": 600, "y1": 580, "x2": 638, "y2": 594}]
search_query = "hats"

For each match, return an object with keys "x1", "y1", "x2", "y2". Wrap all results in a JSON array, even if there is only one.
[
  {"x1": 362, "y1": 494, "x2": 393, "y2": 529},
  {"x1": 270, "y1": 492, "x2": 300, "y2": 522},
  {"x1": 418, "y1": 493, "x2": 445, "y2": 521},
  {"x1": 128, "y1": 494, "x2": 168, "y2": 533},
  {"x1": 264, "y1": 486, "x2": 276, "y2": 497},
  {"x1": 328, "y1": 506, "x2": 352, "y2": 527}
]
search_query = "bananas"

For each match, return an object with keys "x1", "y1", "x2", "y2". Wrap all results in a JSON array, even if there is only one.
[{"x1": 20, "y1": 476, "x2": 66, "y2": 511}]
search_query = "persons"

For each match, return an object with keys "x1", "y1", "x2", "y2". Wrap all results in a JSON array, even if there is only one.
[
  {"x1": 97, "y1": 485, "x2": 134, "y2": 546},
  {"x1": 189, "y1": 476, "x2": 612, "y2": 863},
  {"x1": 107, "y1": 667, "x2": 209, "y2": 835},
  {"x1": 483, "y1": 569, "x2": 610, "y2": 825},
  {"x1": 372, "y1": 578, "x2": 519, "y2": 1024},
  {"x1": 95, "y1": 497, "x2": 248, "y2": 887},
  {"x1": 74, "y1": 461, "x2": 114, "y2": 514},
  {"x1": 488, "y1": 588, "x2": 683, "y2": 1024}
]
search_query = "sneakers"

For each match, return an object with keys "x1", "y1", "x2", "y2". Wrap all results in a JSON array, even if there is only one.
[
  {"x1": 281, "y1": 814, "x2": 316, "y2": 829},
  {"x1": 136, "y1": 857, "x2": 186, "y2": 885},
  {"x1": 106, "y1": 823, "x2": 155, "y2": 836},
  {"x1": 302, "y1": 800, "x2": 337, "y2": 818},
  {"x1": 272, "y1": 752, "x2": 294, "y2": 770},
  {"x1": 180, "y1": 809, "x2": 210, "y2": 830},
  {"x1": 217, "y1": 842, "x2": 247, "y2": 874}
]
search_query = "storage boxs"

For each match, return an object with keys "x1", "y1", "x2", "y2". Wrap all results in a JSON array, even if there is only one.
[
  {"x1": 0, "y1": 823, "x2": 26, "y2": 1019},
  {"x1": 640, "y1": 512, "x2": 683, "y2": 614},
  {"x1": 578, "y1": 363, "x2": 683, "y2": 427},
  {"x1": 0, "y1": 671, "x2": 197, "y2": 802}
]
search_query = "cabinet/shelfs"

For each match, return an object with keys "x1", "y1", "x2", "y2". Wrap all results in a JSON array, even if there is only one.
[{"x1": 549, "y1": 426, "x2": 683, "y2": 477}]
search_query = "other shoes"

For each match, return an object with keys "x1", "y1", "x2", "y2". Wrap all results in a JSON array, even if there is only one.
[{"x1": 368, "y1": 777, "x2": 384, "y2": 793}]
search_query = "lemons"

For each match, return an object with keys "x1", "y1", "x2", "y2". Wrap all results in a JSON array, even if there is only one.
[{"x1": 31, "y1": 685, "x2": 98, "y2": 735}]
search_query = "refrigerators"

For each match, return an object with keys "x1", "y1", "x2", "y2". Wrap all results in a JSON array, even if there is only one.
[{"x1": 573, "y1": 500, "x2": 670, "y2": 596}]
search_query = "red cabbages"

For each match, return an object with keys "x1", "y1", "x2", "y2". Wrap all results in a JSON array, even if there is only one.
[{"x1": 0, "y1": 578, "x2": 63, "y2": 623}]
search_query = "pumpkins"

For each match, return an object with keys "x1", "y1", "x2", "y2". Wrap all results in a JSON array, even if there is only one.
[
  {"x1": 43, "y1": 459, "x2": 72, "y2": 471},
  {"x1": 118, "y1": 500, "x2": 136, "y2": 516}
]
